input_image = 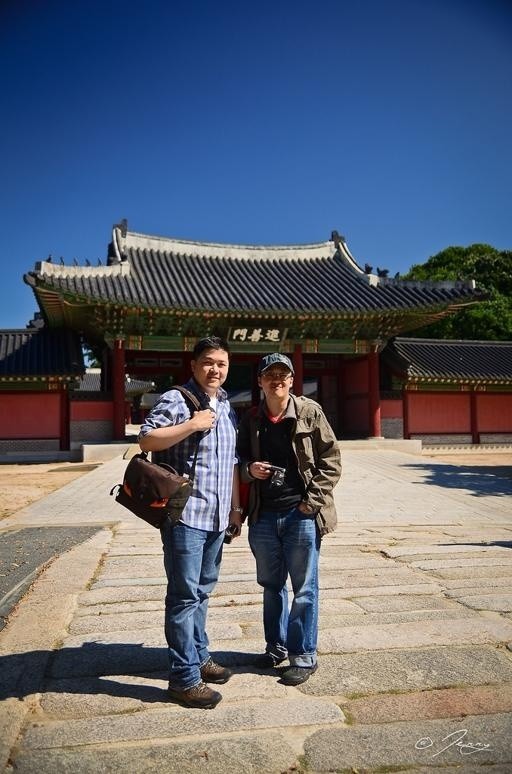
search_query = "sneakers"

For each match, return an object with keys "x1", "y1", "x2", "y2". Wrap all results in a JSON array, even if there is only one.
[
  {"x1": 168, "y1": 683, "x2": 221, "y2": 707},
  {"x1": 202, "y1": 661, "x2": 231, "y2": 684}
]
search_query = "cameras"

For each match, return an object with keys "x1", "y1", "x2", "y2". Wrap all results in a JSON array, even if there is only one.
[{"x1": 261, "y1": 460, "x2": 287, "y2": 487}]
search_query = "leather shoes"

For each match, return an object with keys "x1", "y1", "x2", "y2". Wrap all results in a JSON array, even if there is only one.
[{"x1": 283, "y1": 663, "x2": 317, "y2": 685}]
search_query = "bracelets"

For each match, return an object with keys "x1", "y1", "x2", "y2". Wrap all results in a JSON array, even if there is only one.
[{"x1": 232, "y1": 505, "x2": 244, "y2": 515}]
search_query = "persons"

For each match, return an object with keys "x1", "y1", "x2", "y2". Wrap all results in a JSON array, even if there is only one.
[
  {"x1": 137, "y1": 337, "x2": 245, "y2": 709},
  {"x1": 240, "y1": 352, "x2": 342, "y2": 684}
]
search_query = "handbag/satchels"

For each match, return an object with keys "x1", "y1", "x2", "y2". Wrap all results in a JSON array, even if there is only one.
[{"x1": 116, "y1": 454, "x2": 193, "y2": 528}]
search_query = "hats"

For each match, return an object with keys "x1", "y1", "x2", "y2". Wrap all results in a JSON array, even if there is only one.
[{"x1": 259, "y1": 353, "x2": 294, "y2": 372}]
